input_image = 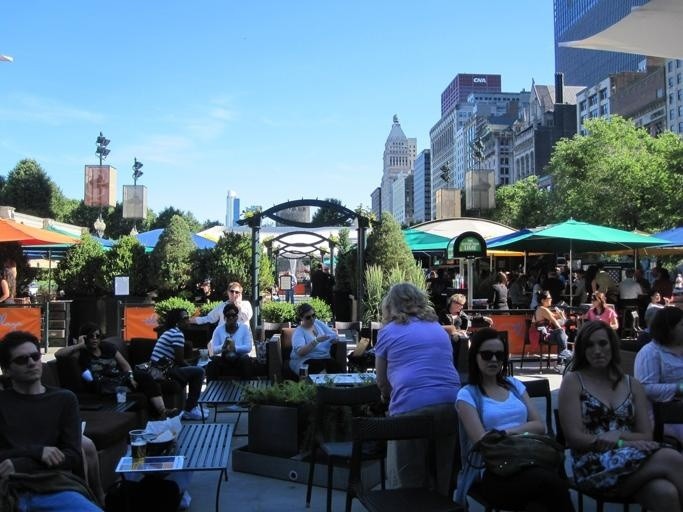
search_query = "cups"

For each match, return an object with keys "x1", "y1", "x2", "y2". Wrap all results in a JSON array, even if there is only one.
[
  {"x1": 129, "y1": 429, "x2": 147, "y2": 459},
  {"x1": 117, "y1": 386, "x2": 127, "y2": 404}
]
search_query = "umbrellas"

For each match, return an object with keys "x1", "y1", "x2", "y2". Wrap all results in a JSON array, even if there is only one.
[
  {"x1": 645, "y1": 225, "x2": 682, "y2": 247},
  {"x1": 22, "y1": 225, "x2": 82, "y2": 294},
  {"x1": 486, "y1": 225, "x2": 552, "y2": 294},
  {"x1": 0, "y1": 217, "x2": 85, "y2": 245},
  {"x1": 505, "y1": 216, "x2": 673, "y2": 308},
  {"x1": 591, "y1": 228, "x2": 682, "y2": 281}
]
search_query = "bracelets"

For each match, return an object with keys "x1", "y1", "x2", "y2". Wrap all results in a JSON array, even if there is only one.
[
  {"x1": 618, "y1": 440, "x2": 622, "y2": 447},
  {"x1": 524, "y1": 432, "x2": 528, "y2": 435},
  {"x1": 127, "y1": 371, "x2": 133, "y2": 379},
  {"x1": 315, "y1": 338, "x2": 320, "y2": 344}
]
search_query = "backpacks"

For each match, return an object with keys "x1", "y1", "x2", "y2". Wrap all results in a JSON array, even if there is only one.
[{"x1": 104, "y1": 478, "x2": 181, "y2": 512}]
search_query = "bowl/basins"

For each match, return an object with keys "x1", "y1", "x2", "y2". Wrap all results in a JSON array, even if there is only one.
[{"x1": 142, "y1": 433, "x2": 176, "y2": 456}]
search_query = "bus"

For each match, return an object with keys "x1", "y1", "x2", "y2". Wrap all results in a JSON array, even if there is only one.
[{"x1": 446, "y1": 250, "x2": 558, "y2": 277}]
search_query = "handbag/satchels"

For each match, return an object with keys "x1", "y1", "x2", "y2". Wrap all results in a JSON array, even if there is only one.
[
  {"x1": 466, "y1": 429, "x2": 566, "y2": 487},
  {"x1": 135, "y1": 357, "x2": 175, "y2": 386}
]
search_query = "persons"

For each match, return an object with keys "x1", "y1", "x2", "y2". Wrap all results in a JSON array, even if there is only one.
[
  {"x1": 373, "y1": 283, "x2": 461, "y2": 414},
  {"x1": 1, "y1": 260, "x2": 17, "y2": 304},
  {"x1": 54, "y1": 323, "x2": 180, "y2": 418},
  {"x1": 434, "y1": 259, "x2": 683, "y2": 374},
  {"x1": 0, "y1": 331, "x2": 104, "y2": 512},
  {"x1": 285, "y1": 271, "x2": 297, "y2": 304},
  {"x1": 186, "y1": 280, "x2": 257, "y2": 359},
  {"x1": 558, "y1": 319, "x2": 682, "y2": 512},
  {"x1": 455, "y1": 327, "x2": 574, "y2": 511},
  {"x1": 290, "y1": 303, "x2": 339, "y2": 376},
  {"x1": 151, "y1": 309, "x2": 210, "y2": 421},
  {"x1": 632, "y1": 307, "x2": 682, "y2": 444},
  {"x1": 311, "y1": 264, "x2": 336, "y2": 304}
]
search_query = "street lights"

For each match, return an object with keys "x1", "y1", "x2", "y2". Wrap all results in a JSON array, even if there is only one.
[
  {"x1": 469, "y1": 134, "x2": 491, "y2": 219},
  {"x1": 437, "y1": 157, "x2": 454, "y2": 220},
  {"x1": 89, "y1": 132, "x2": 110, "y2": 240},
  {"x1": 125, "y1": 155, "x2": 142, "y2": 238}
]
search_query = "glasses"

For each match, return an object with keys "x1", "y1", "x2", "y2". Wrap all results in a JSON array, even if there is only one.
[
  {"x1": 305, "y1": 313, "x2": 316, "y2": 320},
  {"x1": 183, "y1": 315, "x2": 190, "y2": 321},
  {"x1": 11, "y1": 352, "x2": 42, "y2": 365},
  {"x1": 231, "y1": 289, "x2": 239, "y2": 294},
  {"x1": 480, "y1": 350, "x2": 506, "y2": 361},
  {"x1": 88, "y1": 334, "x2": 101, "y2": 339}
]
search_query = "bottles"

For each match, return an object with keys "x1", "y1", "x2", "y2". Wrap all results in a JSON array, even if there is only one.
[
  {"x1": 453, "y1": 274, "x2": 464, "y2": 290},
  {"x1": 554, "y1": 306, "x2": 566, "y2": 320},
  {"x1": 314, "y1": 376, "x2": 321, "y2": 384},
  {"x1": 365, "y1": 368, "x2": 376, "y2": 384},
  {"x1": 675, "y1": 273, "x2": 682, "y2": 289}
]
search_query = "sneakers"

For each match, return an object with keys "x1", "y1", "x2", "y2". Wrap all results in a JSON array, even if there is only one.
[
  {"x1": 559, "y1": 349, "x2": 571, "y2": 359},
  {"x1": 183, "y1": 407, "x2": 209, "y2": 420},
  {"x1": 552, "y1": 363, "x2": 565, "y2": 374},
  {"x1": 196, "y1": 403, "x2": 209, "y2": 414},
  {"x1": 160, "y1": 407, "x2": 179, "y2": 420}
]
